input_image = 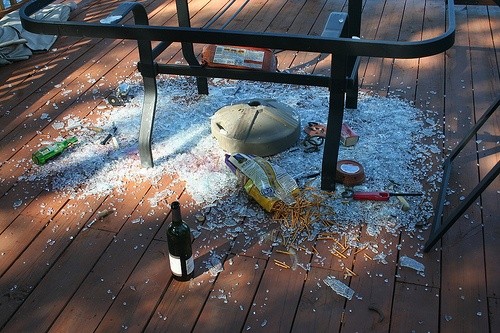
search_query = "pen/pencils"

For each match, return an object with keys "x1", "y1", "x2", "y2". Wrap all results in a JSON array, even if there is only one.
[
  {"x1": 296, "y1": 173, "x2": 320, "y2": 182},
  {"x1": 101, "y1": 126, "x2": 117, "y2": 145}
]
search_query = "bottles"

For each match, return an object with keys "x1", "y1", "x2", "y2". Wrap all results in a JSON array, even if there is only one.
[
  {"x1": 32, "y1": 136, "x2": 78, "y2": 165},
  {"x1": 166, "y1": 200, "x2": 195, "y2": 282}
]
